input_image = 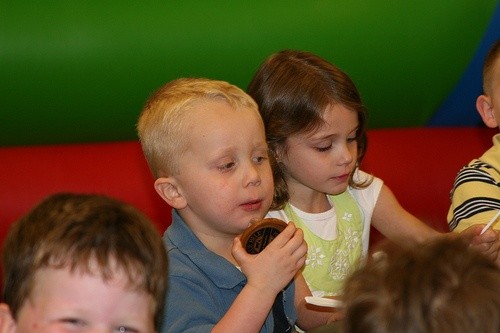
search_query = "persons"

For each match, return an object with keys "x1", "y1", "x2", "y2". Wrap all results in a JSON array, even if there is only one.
[
  {"x1": 446, "y1": 39, "x2": 500, "y2": 233},
  {"x1": 337, "y1": 231, "x2": 500, "y2": 333},
  {"x1": 0, "y1": 189, "x2": 167, "y2": 333},
  {"x1": 243, "y1": 49, "x2": 500, "y2": 333},
  {"x1": 137, "y1": 76, "x2": 308, "y2": 333}
]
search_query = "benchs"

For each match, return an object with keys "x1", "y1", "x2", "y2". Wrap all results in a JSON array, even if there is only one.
[{"x1": 0, "y1": 123, "x2": 500, "y2": 306}]
